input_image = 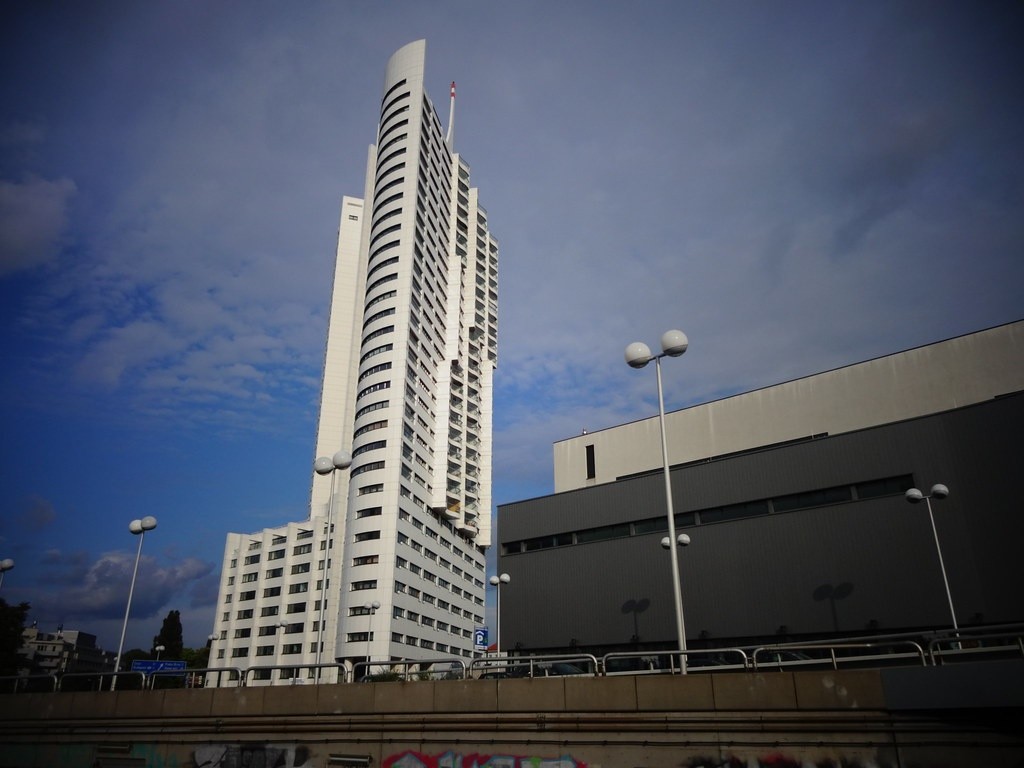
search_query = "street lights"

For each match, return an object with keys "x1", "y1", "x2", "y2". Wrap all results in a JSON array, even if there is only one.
[
  {"x1": 488, "y1": 572, "x2": 510, "y2": 673},
  {"x1": 271, "y1": 619, "x2": 288, "y2": 686},
  {"x1": 150, "y1": 645, "x2": 166, "y2": 690},
  {"x1": 662, "y1": 532, "x2": 692, "y2": 662},
  {"x1": 206, "y1": 633, "x2": 219, "y2": 688},
  {"x1": 364, "y1": 601, "x2": 381, "y2": 682},
  {"x1": 315, "y1": 451, "x2": 353, "y2": 687},
  {"x1": 0, "y1": 556, "x2": 14, "y2": 588},
  {"x1": 109, "y1": 516, "x2": 157, "y2": 691},
  {"x1": 625, "y1": 326, "x2": 690, "y2": 675},
  {"x1": 905, "y1": 482, "x2": 963, "y2": 652}
]
